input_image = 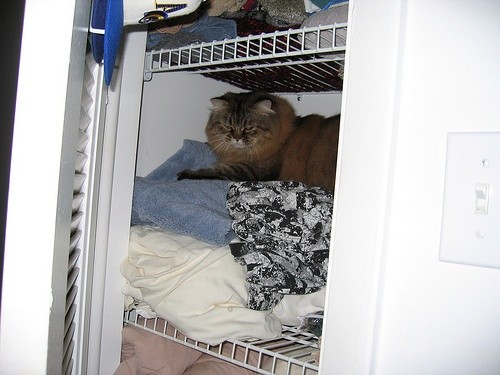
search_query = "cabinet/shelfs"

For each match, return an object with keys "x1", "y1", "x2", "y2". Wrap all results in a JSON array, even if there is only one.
[{"x1": 121, "y1": 1, "x2": 350, "y2": 373}]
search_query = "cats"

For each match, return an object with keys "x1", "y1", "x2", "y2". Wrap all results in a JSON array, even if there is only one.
[{"x1": 177, "y1": 88, "x2": 341, "y2": 195}]
